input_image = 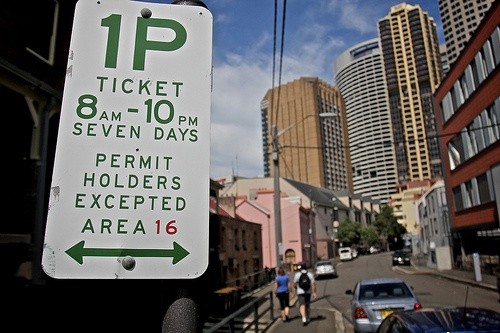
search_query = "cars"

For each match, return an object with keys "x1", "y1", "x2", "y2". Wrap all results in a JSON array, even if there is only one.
[
  {"x1": 315, "y1": 260, "x2": 336, "y2": 280},
  {"x1": 351, "y1": 249, "x2": 358, "y2": 258},
  {"x1": 369, "y1": 245, "x2": 381, "y2": 254},
  {"x1": 345, "y1": 279, "x2": 421, "y2": 333},
  {"x1": 375, "y1": 307, "x2": 500, "y2": 333},
  {"x1": 391, "y1": 250, "x2": 410, "y2": 266}
]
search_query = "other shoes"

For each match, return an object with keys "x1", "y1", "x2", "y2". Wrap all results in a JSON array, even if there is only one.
[{"x1": 283, "y1": 315, "x2": 289, "y2": 322}]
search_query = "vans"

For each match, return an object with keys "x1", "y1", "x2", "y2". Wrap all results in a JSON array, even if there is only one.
[{"x1": 339, "y1": 247, "x2": 353, "y2": 261}]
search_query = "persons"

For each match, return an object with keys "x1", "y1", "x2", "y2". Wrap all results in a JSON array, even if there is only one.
[
  {"x1": 293, "y1": 262, "x2": 316, "y2": 325},
  {"x1": 274, "y1": 269, "x2": 294, "y2": 322}
]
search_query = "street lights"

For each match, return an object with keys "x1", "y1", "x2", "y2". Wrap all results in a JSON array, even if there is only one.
[{"x1": 270, "y1": 112, "x2": 339, "y2": 276}]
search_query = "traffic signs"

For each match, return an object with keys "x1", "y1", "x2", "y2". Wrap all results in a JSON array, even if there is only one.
[{"x1": 42, "y1": 0, "x2": 212, "y2": 278}]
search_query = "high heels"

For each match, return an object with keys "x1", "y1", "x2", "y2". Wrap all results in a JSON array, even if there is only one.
[{"x1": 302, "y1": 321, "x2": 309, "y2": 327}]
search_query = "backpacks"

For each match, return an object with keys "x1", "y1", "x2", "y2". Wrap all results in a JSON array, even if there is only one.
[{"x1": 299, "y1": 270, "x2": 311, "y2": 291}]
